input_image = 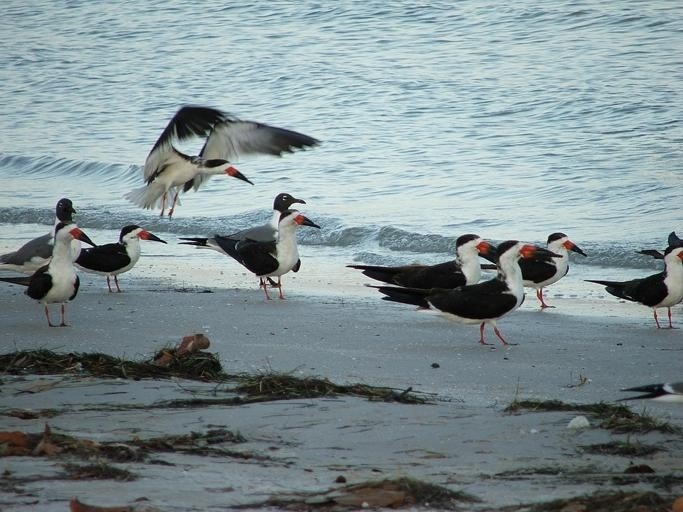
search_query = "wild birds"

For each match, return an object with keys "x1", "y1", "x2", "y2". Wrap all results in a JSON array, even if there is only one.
[
  {"x1": 122, "y1": 105, "x2": 323, "y2": 221},
  {"x1": 344, "y1": 229, "x2": 586, "y2": 346},
  {"x1": 214, "y1": 209, "x2": 321, "y2": 301},
  {"x1": 0, "y1": 221, "x2": 97, "y2": 327},
  {"x1": 0, "y1": 198, "x2": 81, "y2": 277},
  {"x1": 612, "y1": 381, "x2": 683, "y2": 405},
  {"x1": 177, "y1": 192, "x2": 306, "y2": 289},
  {"x1": 585, "y1": 231, "x2": 683, "y2": 332},
  {"x1": 72, "y1": 224, "x2": 168, "y2": 293}
]
